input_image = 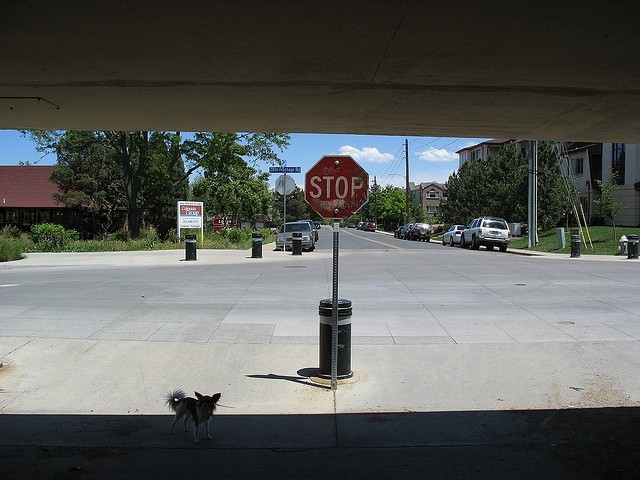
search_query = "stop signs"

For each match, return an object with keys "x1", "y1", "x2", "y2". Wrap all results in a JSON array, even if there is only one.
[{"x1": 304, "y1": 155, "x2": 369, "y2": 222}]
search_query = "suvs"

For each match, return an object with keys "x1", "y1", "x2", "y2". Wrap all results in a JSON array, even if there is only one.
[{"x1": 459, "y1": 216, "x2": 511, "y2": 252}]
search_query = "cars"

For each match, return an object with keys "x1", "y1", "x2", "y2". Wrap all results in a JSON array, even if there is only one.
[
  {"x1": 394, "y1": 223, "x2": 431, "y2": 242},
  {"x1": 442, "y1": 224, "x2": 468, "y2": 247},
  {"x1": 276, "y1": 221, "x2": 316, "y2": 251},
  {"x1": 349, "y1": 222, "x2": 376, "y2": 232},
  {"x1": 297, "y1": 220, "x2": 318, "y2": 242}
]
